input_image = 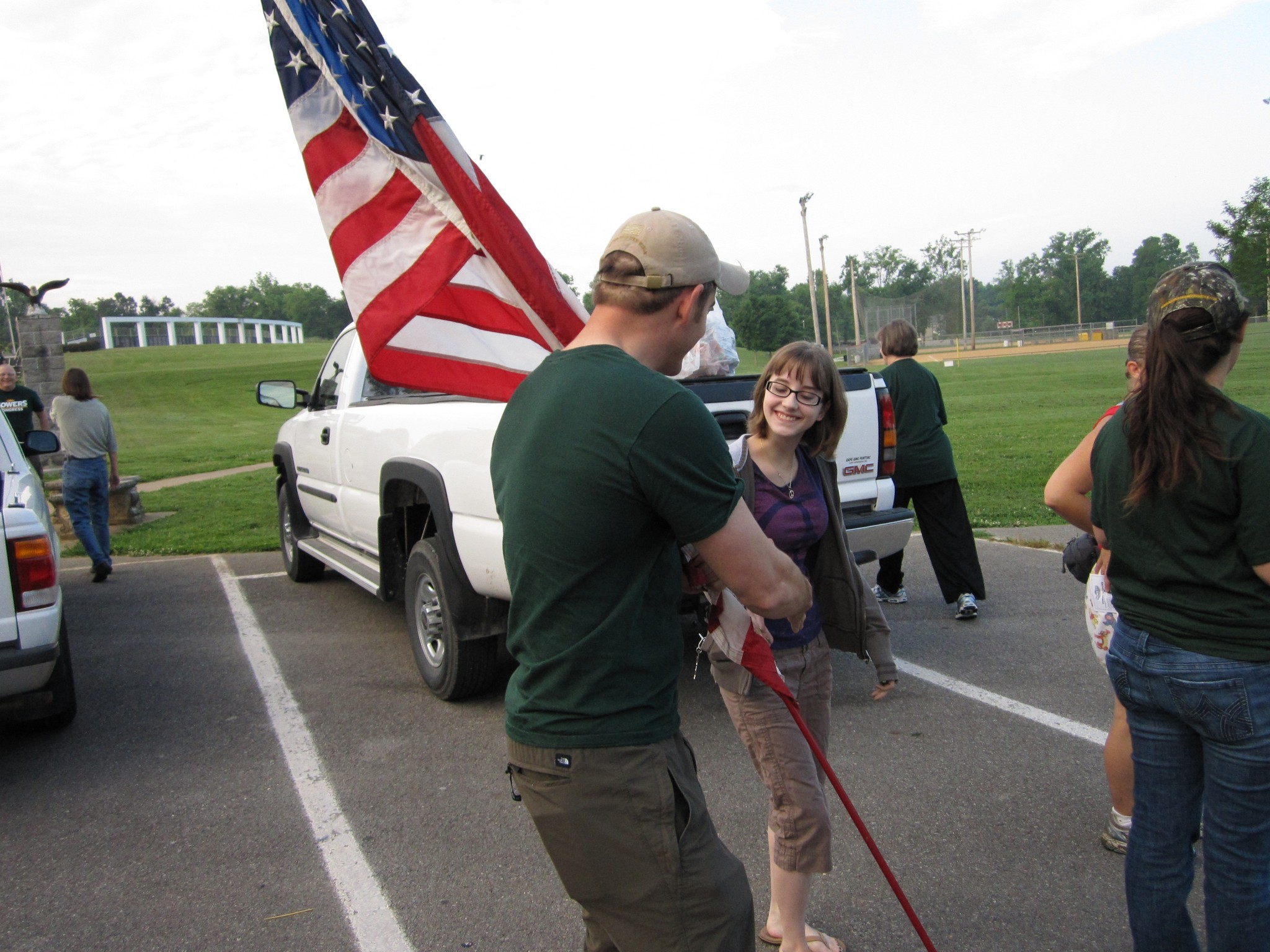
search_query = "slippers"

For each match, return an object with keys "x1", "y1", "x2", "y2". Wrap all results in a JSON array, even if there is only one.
[{"x1": 759, "y1": 924, "x2": 846, "y2": 952}]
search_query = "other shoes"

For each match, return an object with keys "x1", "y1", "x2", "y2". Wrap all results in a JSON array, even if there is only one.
[
  {"x1": 88, "y1": 560, "x2": 109, "y2": 572},
  {"x1": 92, "y1": 563, "x2": 114, "y2": 582}
]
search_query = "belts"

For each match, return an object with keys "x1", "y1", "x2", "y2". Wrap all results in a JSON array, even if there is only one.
[{"x1": 64, "y1": 456, "x2": 97, "y2": 461}]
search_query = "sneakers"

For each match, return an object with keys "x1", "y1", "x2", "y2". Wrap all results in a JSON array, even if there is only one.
[
  {"x1": 955, "y1": 592, "x2": 978, "y2": 618},
  {"x1": 869, "y1": 584, "x2": 907, "y2": 603},
  {"x1": 1102, "y1": 818, "x2": 1196, "y2": 856}
]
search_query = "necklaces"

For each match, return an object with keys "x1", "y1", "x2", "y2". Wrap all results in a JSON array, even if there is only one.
[{"x1": 760, "y1": 438, "x2": 795, "y2": 498}]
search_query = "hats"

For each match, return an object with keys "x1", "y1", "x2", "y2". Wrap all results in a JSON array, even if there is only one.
[
  {"x1": 598, "y1": 206, "x2": 750, "y2": 296},
  {"x1": 1146, "y1": 262, "x2": 1250, "y2": 343}
]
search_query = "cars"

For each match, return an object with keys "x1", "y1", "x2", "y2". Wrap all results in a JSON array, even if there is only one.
[{"x1": 0, "y1": 396, "x2": 87, "y2": 732}]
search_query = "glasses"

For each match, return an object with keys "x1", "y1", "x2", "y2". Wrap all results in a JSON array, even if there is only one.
[{"x1": 765, "y1": 380, "x2": 827, "y2": 407}]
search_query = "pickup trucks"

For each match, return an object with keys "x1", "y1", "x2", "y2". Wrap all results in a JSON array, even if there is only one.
[{"x1": 251, "y1": 316, "x2": 920, "y2": 703}]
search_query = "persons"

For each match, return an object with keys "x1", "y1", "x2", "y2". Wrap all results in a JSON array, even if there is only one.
[
  {"x1": 1043, "y1": 261, "x2": 1270, "y2": 951},
  {"x1": 490, "y1": 208, "x2": 813, "y2": 952},
  {"x1": 871, "y1": 319, "x2": 986, "y2": 620},
  {"x1": 49, "y1": 368, "x2": 120, "y2": 582},
  {"x1": 700, "y1": 341, "x2": 899, "y2": 952},
  {"x1": 0, "y1": 363, "x2": 49, "y2": 492}
]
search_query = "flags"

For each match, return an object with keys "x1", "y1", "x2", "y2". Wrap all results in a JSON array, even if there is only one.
[{"x1": 261, "y1": 0, "x2": 801, "y2": 710}]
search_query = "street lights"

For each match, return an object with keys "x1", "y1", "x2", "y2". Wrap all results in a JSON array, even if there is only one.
[
  {"x1": 813, "y1": 232, "x2": 838, "y2": 357},
  {"x1": 798, "y1": 190, "x2": 824, "y2": 345}
]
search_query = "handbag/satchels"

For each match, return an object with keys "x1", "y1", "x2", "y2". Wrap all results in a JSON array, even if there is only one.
[
  {"x1": 686, "y1": 298, "x2": 740, "y2": 377},
  {"x1": 1061, "y1": 533, "x2": 1101, "y2": 584}
]
